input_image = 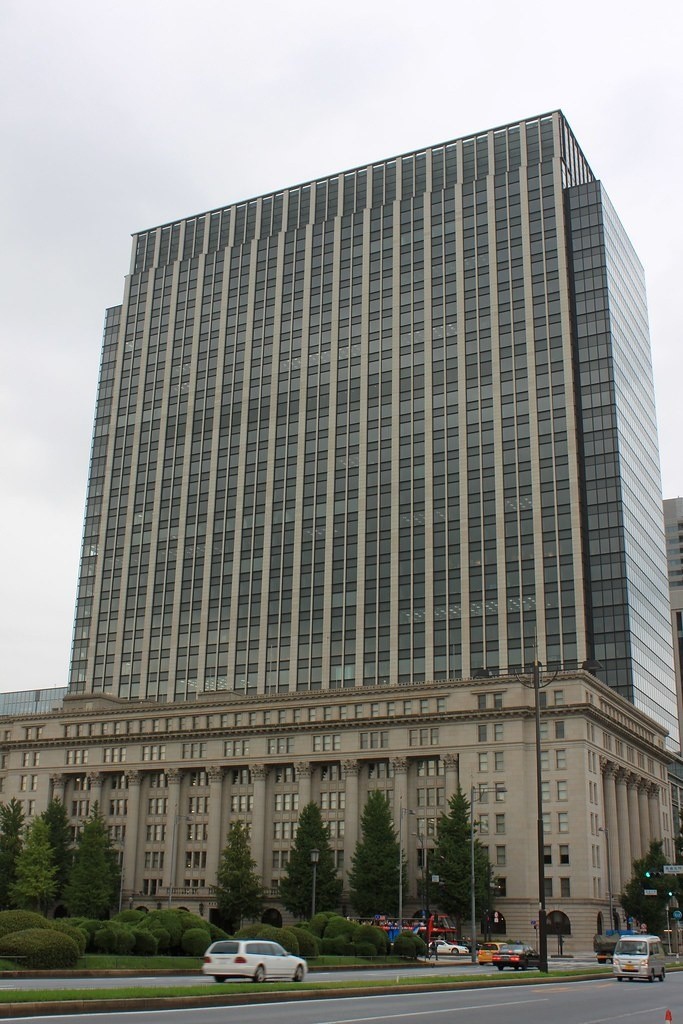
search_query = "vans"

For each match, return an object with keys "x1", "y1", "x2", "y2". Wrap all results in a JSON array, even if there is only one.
[{"x1": 612, "y1": 935, "x2": 666, "y2": 982}]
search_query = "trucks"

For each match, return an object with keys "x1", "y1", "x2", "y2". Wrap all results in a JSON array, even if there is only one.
[{"x1": 593, "y1": 929, "x2": 635, "y2": 964}]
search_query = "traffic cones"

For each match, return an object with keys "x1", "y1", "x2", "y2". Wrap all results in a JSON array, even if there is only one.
[{"x1": 665, "y1": 1009, "x2": 672, "y2": 1024}]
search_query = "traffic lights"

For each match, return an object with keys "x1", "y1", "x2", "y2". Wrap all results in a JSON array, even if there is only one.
[
  {"x1": 484, "y1": 905, "x2": 491, "y2": 925},
  {"x1": 645, "y1": 871, "x2": 663, "y2": 879},
  {"x1": 668, "y1": 891, "x2": 680, "y2": 896}
]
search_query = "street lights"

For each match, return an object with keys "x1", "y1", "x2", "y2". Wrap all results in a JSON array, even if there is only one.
[
  {"x1": 398, "y1": 795, "x2": 415, "y2": 934},
  {"x1": 471, "y1": 785, "x2": 508, "y2": 966},
  {"x1": 311, "y1": 848, "x2": 320, "y2": 917},
  {"x1": 473, "y1": 660, "x2": 604, "y2": 973},
  {"x1": 169, "y1": 804, "x2": 193, "y2": 909}
]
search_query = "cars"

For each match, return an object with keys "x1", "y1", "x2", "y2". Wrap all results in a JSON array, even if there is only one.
[
  {"x1": 478, "y1": 942, "x2": 507, "y2": 965},
  {"x1": 492, "y1": 945, "x2": 540, "y2": 970},
  {"x1": 204, "y1": 940, "x2": 308, "y2": 983},
  {"x1": 428, "y1": 940, "x2": 469, "y2": 955}
]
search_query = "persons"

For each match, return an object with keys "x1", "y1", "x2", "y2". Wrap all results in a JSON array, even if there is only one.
[{"x1": 429, "y1": 939, "x2": 439, "y2": 960}]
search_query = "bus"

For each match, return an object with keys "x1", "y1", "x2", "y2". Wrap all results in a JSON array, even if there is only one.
[{"x1": 347, "y1": 914, "x2": 458, "y2": 945}]
